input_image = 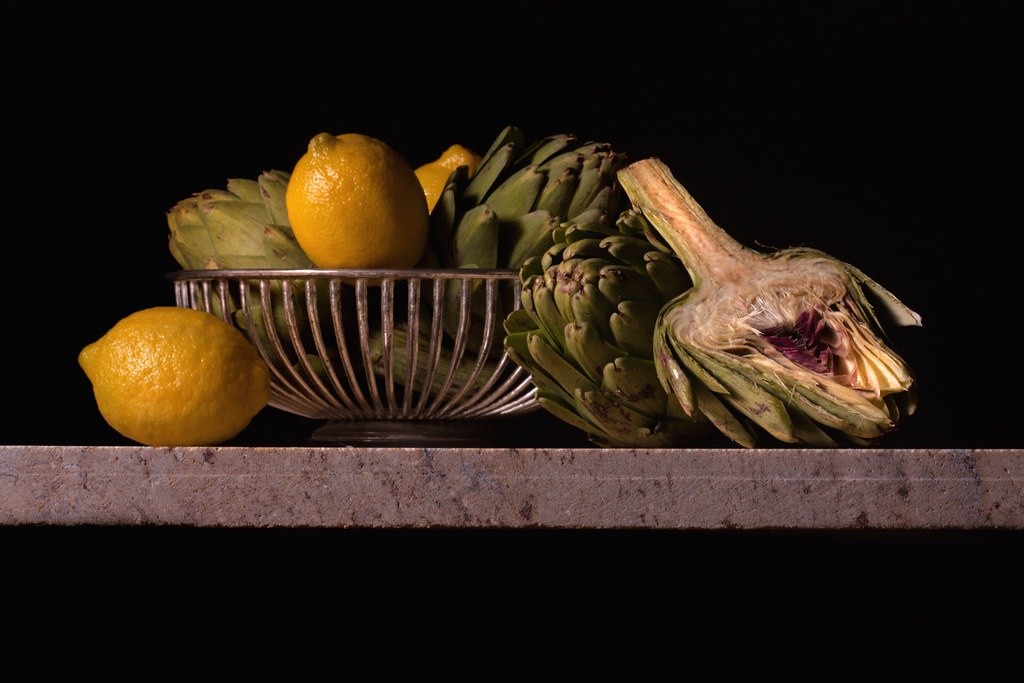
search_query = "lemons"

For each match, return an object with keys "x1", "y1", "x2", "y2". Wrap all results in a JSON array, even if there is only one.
[
  {"x1": 285, "y1": 132, "x2": 429, "y2": 287},
  {"x1": 411, "y1": 144, "x2": 483, "y2": 215},
  {"x1": 78, "y1": 307, "x2": 274, "y2": 447}
]
